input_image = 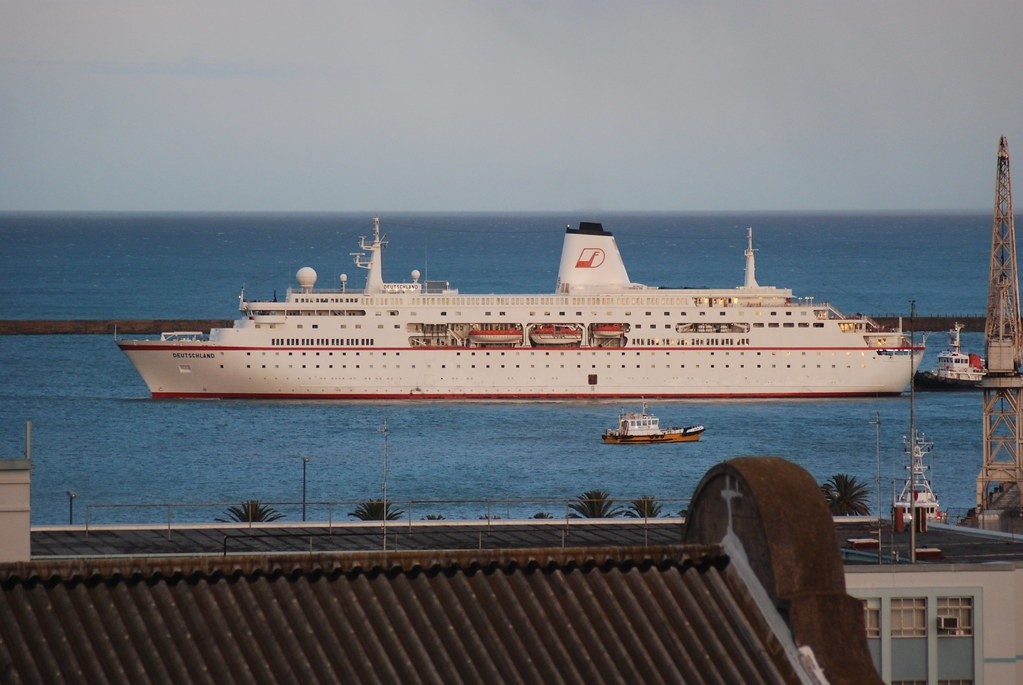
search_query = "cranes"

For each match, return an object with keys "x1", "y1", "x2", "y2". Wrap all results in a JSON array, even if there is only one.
[{"x1": 975, "y1": 135, "x2": 1023, "y2": 512}]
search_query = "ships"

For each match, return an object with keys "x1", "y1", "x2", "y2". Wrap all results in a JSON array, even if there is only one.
[{"x1": 116, "y1": 217, "x2": 934, "y2": 399}]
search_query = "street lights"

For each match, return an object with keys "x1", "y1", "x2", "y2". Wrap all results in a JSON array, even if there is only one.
[
  {"x1": 906, "y1": 298, "x2": 920, "y2": 562},
  {"x1": 378, "y1": 420, "x2": 389, "y2": 552},
  {"x1": 868, "y1": 412, "x2": 883, "y2": 563},
  {"x1": 302, "y1": 456, "x2": 309, "y2": 522},
  {"x1": 66, "y1": 489, "x2": 76, "y2": 525}
]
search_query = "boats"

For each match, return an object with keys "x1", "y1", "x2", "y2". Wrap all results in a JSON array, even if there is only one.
[
  {"x1": 531, "y1": 324, "x2": 583, "y2": 346},
  {"x1": 935, "y1": 322, "x2": 987, "y2": 388},
  {"x1": 890, "y1": 430, "x2": 946, "y2": 520},
  {"x1": 591, "y1": 325, "x2": 625, "y2": 340},
  {"x1": 468, "y1": 329, "x2": 521, "y2": 344},
  {"x1": 601, "y1": 394, "x2": 706, "y2": 443}
]
